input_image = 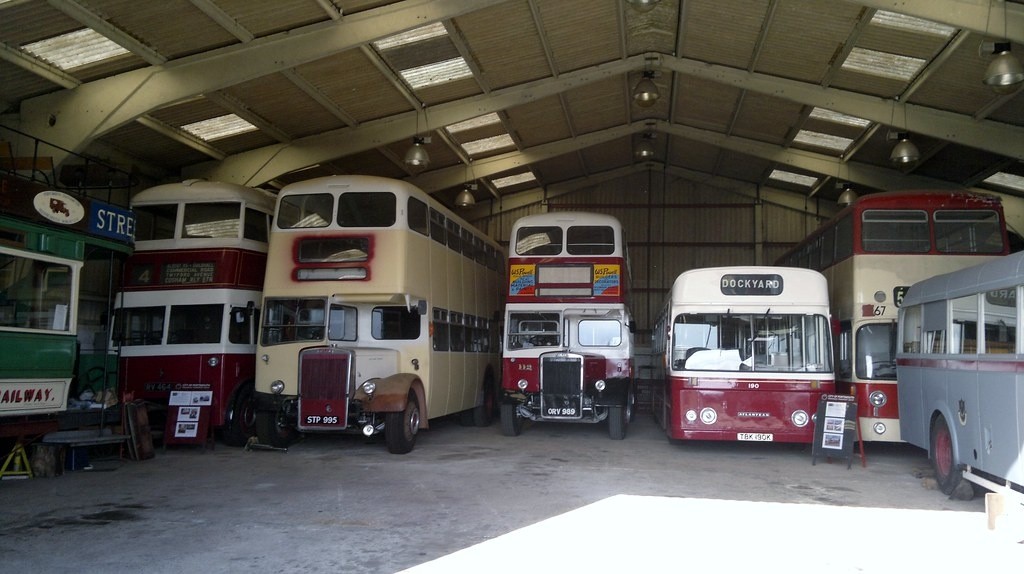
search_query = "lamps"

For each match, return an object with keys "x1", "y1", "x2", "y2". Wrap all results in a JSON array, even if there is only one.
[
  {"x1": 633, "y1": 66, "x2": 660, "y2": 101},
  {"x1": 837, "y1": 162, "x2": 858, "y2": 206},
  {"x1": 455, "y1": 167, "x2": 475, "y2": 206},
  {"x1": 405, "y1": 112, "x2": 432, "y2": 166},
  {"x1": 634, "y1": 133, "x2": 655, "y2": 157},
  {"x1": 984, "y1": 0, "x2": 1024, "y2": 85},
  {"x1": 889, "y1": 101, "x2": 921, "y2": 163}
]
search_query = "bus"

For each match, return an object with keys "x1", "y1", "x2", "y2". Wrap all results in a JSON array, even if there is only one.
[
  {"x1": 500, "y1": 211, "x2": 638, "y2": 440},
  {"x1": 111, "y1": 178, "x2": 311, "y2": 447},
  {"x1": 895, "y1": 249, "x2": 1024, "y2": 506},
  {"x1": 773, "y1": 189, "x2": 1012, "y2": 444},
  {"x1": 650, "y1": 264, "x2": 836, "y2": 444},
  {"x1": 254, "y1": 175, "x2": 505, "y2": 455},
  {"x1": 0, "y1": 164, "x2": 138, "y2": 441}
]
